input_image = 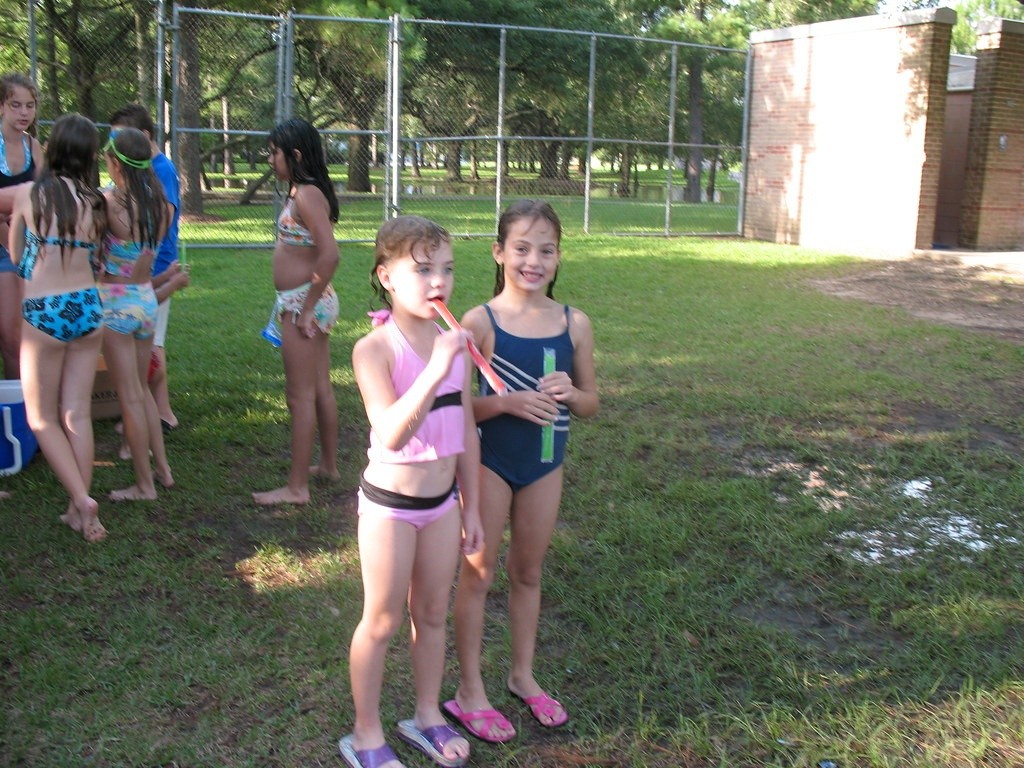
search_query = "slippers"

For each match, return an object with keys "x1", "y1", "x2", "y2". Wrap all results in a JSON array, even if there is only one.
[
  {"x1": 397, "y1": 719, "x2": 471, "y2": 768},
  {"x1": 339, "y1": 733, "x2": 407, "y2": 768},
  {"x1": 443, "y1": 699, "x2": 517, "y2": 743},
  {"x1": 507, "y1": 676, "x2": 569, "y2": 728}
]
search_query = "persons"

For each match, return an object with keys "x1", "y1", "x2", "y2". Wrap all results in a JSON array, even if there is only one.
[
  {"x1": 251, "y1": 116, "x2": 342, "y2": 507},
  {"x1": 339, "y1": 213, "x2": 484, "y2": 768},
  {"x1": 109, "y1": 103, "x2": 184, "y2": 435},
  {"x1": 0, "y1": 70, "x2": 44, "y2": 382},
  {"x1": 8, "y1": 112, "x2": 110, "y2": 543},
  {"x1": 94, "y1": 127, "x2": 174, "y2": 501},
  {"x1": 443, "y1": 198, "x2": 600, "y2": 739}
]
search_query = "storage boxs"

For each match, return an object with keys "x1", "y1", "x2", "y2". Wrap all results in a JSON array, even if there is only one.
[
  {"x1": 1, "y1": 379, "x2": 41, "y2": 477},
  {"x1": 89, "y1": 356, "x2": 124, "y2": 420}
]
